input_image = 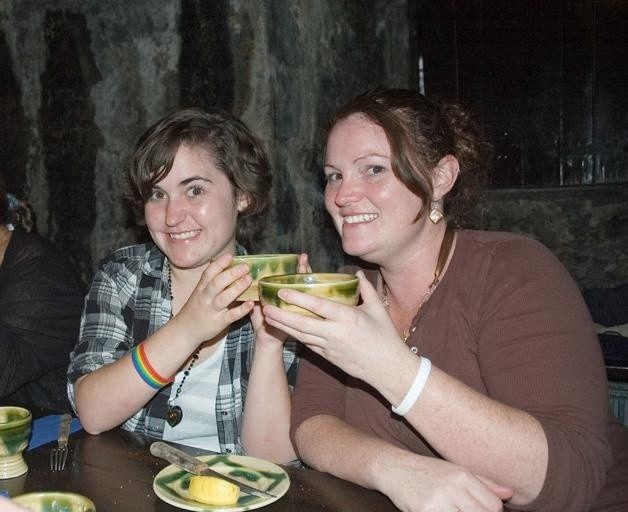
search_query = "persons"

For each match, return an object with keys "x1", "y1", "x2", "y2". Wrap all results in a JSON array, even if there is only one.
[
  {"x1": 0, "y1": 174, "x2": 90, "y2": 417},
  {"x1": 262, "y1": 85, "x2": 628, "y2": 512},
  {"x1": 66, "y1": 104, "x2": 308, "y2": 470}
]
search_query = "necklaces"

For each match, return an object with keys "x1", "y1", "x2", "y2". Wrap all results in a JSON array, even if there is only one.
[
  {"x1": 378, "y1": 227, "x2": 455, "y2": 344},
  {"x1": 160, "y1": 249, "x2": 242, "y2": 428}
]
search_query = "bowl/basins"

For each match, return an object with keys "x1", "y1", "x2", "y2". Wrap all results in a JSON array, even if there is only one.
[
  {"x1": 12, "y1": 491, "x2": 96, "y2": 512},
  {"x1": 0, "y1": 403, "x2": 35, "y2": 481},
  {"x1": 258, "y1": 273, "x2": 361, "y2": 326},
  {"x1": 209, "y1": 252, "x2": 299, "y2": 301}
]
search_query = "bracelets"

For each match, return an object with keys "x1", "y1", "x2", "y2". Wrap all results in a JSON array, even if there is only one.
[
  {"x1": 392, "y1": 355, "x2": 431, "y2": 416},
  {"x1": 131, "y1": 343, "x2": 175, "y2": 391}
]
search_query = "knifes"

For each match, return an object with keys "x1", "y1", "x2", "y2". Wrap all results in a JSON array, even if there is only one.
[{"x1": 149, "y1": 440, "x2": 277, "y2": 499}]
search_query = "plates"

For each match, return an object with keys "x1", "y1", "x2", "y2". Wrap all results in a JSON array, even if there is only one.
[{"x1": 152, "y1": 454, "x2": 290, "y2": 512}]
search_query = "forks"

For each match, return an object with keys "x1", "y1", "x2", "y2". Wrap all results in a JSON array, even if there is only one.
[{"x1": 50, "y1": 413, "x2": 77, "y2": 473}]
